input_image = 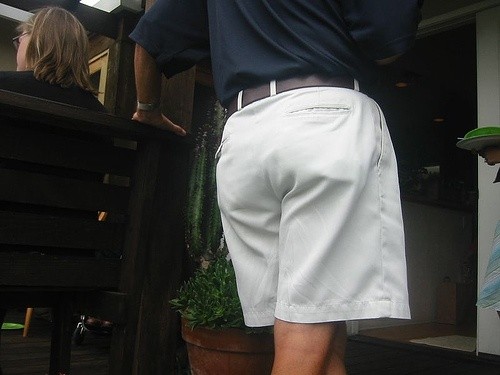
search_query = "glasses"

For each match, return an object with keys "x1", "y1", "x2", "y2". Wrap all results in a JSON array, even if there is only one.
[{"x1": 12, "y1": 31, "x2": 31, "y2": 49}]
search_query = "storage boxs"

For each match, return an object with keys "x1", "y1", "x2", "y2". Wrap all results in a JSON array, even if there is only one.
[{"x1": 435, "y1": 281, "x2": 465, "y2": 324}]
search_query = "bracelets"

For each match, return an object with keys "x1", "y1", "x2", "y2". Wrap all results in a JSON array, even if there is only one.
[{"x1": 136, "y1": 100, "x2": 162, "y2": 113}]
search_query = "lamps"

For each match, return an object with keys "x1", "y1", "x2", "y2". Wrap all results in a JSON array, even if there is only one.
[{"x1": 392, "y1": 68, "x2": 421, "y2": 89}]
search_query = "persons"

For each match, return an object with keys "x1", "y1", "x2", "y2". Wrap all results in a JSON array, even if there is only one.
[
  {"x1": 0, "y1": 6, "x2": 113, "y2": 334},
  {"x1": 129, "y1": 0, "x2": 411, "y2": 374},
  {"x1": 476, "y1": 144, "x2": 500, "y2": 319}
]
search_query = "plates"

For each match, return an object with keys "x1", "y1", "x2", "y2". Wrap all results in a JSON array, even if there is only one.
[
  {"x1": 464, "y1": 127, "x2": 500, "y2": 140},
  {"x1": 456, "y1": 134, "x2": 500, "y2": 150}
]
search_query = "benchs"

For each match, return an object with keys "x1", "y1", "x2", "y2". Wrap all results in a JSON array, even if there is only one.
[{"x1": 0, "y1": 89, "x2": 194, "y2": 375}]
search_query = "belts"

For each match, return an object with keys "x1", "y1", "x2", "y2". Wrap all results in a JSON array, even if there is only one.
[{"x1": 226, "y1": 71, "x2": 353, "y2": 112}]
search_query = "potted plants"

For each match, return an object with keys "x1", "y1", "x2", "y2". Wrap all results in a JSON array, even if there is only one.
[{"x1": 168, "y1": 96, "x2": 275, "y2": 375}]
